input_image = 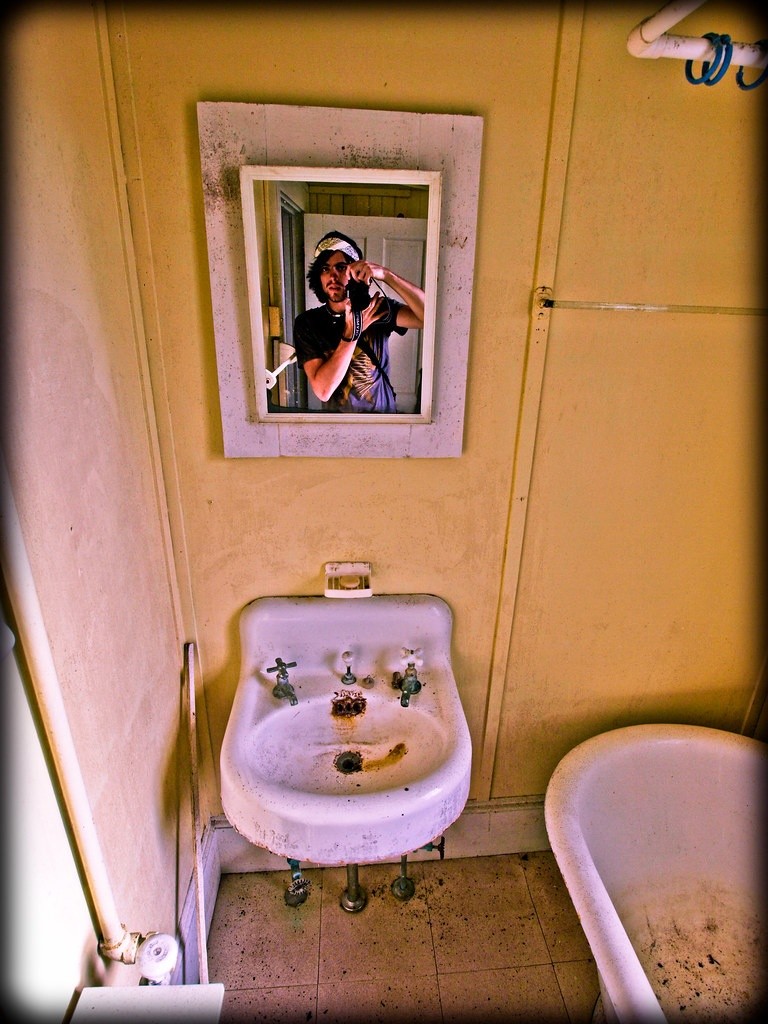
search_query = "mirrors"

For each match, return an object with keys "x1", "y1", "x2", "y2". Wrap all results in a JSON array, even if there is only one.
[{"x1": 239, "y1": 165, "x2": 442, "y2": 425}]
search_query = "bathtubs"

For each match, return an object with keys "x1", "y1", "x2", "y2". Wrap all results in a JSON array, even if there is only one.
[{"x1": 542, "y1": 723, "x2": 768, "y2": 1024}]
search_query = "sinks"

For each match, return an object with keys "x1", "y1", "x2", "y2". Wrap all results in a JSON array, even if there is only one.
[{"x1": 219, "y1": 681, "x2": 475, "y2": 866}]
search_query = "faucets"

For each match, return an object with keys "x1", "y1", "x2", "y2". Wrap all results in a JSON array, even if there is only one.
[
  {"x1": 266, "y1": 657, "x2": 298, "y2": 706},
  {"x1": 391, "y1": 646, "x2": 422, "y2": 708}
]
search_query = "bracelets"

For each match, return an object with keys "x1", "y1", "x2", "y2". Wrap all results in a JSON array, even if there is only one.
[{"x1": 341, "y1": 335, "x2": 352, "y2": 342}]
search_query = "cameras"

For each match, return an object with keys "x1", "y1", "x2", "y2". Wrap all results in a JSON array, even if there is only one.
[{"x1": 345, "y1": 271, "x2": 370, "y2": 312}]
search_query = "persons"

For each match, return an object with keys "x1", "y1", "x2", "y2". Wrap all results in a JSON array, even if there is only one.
[{"x1": 294, "y1": 231, "x2": 425, "y2": 413}]
